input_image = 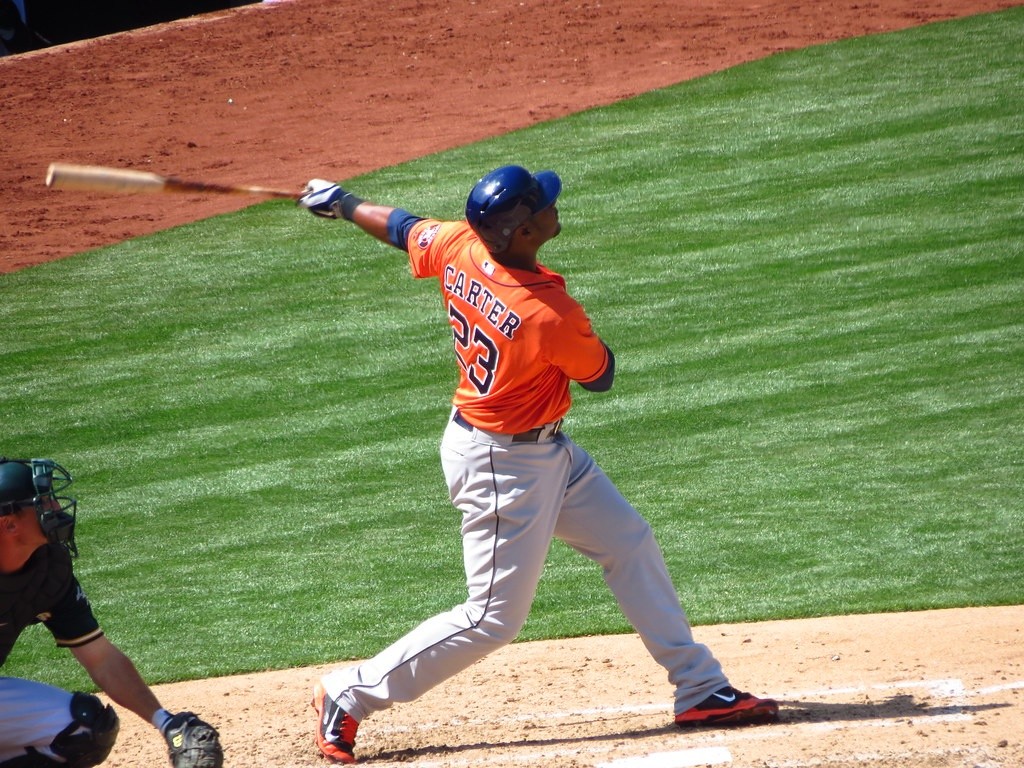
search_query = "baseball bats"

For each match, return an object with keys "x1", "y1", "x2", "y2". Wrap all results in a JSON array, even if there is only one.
[{"x1": 46, "y1": 161, "x2": 308, "y2": 200}]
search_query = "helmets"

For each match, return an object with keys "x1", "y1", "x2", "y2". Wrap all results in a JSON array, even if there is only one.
[
  {"x1": 466, "y1": 166, "x2": 562, "y2": 253},
  {"x1": 0, "y1": 459, "x2": 43, "y2": 511}
]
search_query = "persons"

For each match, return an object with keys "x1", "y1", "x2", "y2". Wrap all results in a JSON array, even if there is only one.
[
  {"x1": 299, "y1": 164, "x2": 780, "y2": 766},
  {"x1": 0, "y1": 456, "x2": 226, "y2": 768}
]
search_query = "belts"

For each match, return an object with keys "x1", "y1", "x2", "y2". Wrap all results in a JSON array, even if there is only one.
[{"x1": 456, "y1": 411, "x2": 565, "y2": 443}]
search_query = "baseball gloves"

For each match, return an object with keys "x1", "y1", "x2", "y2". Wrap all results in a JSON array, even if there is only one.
[{"x1": 164, "y1": 710, "x2": 222, "y2": 768}]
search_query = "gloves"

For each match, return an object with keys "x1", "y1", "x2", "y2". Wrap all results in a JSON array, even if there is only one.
[{"x1": 298, "y1": 178, "x2": 360, "y2": 220}]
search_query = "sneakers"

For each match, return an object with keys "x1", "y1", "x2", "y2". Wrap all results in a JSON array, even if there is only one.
[
  {"x1": 313, "y1": 679, "x2": 358, "y2": 764},
  {"x1": 675, "y1": 686, "x2": 779, "y2": 726}
]
